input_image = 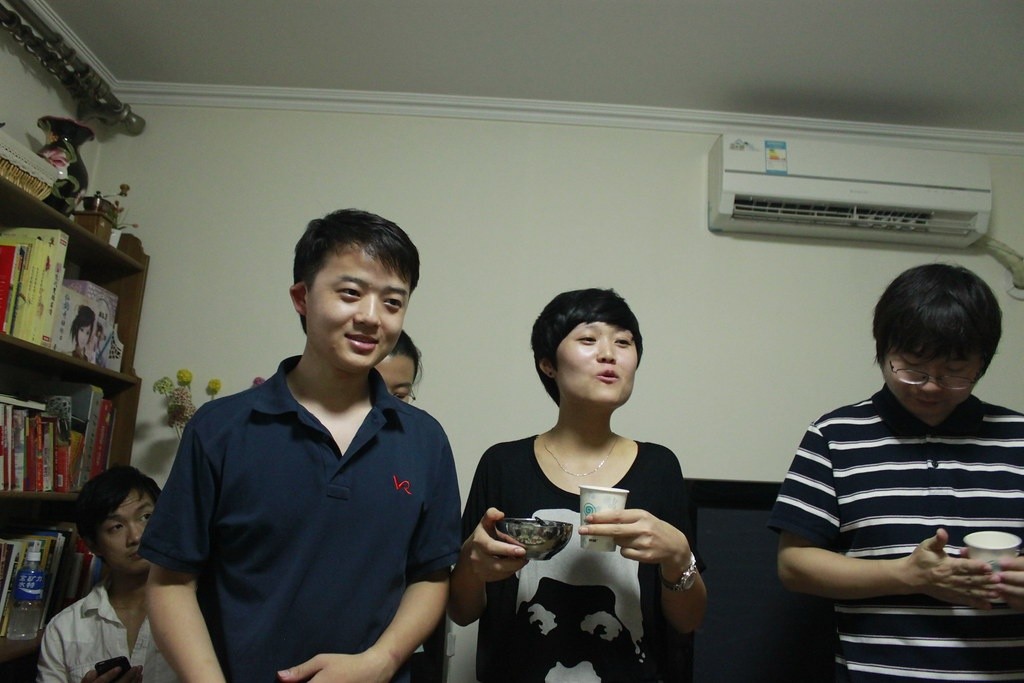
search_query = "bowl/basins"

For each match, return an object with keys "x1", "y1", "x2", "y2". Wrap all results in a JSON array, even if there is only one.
[
  {"x1": 494, "y1": 518, "x2": 574, "y2": 560},
  {"x1": 81, "y1": 190, "x2": 112, "y2": 215}
]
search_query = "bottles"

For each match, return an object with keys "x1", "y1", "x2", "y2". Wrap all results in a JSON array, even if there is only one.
[
  {"x1": 34, "y1": 115, "x2": 89, "y2": 217},
  {"x1": 7, "y1": 551, "x2": 49, "y2": 640}
]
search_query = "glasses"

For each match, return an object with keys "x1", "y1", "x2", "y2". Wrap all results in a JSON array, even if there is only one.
[
  {"x1": 392, "y1": 388, "x2": 416, "y2": 405},
  {"x1": 885, "y1": 348, "x2": 984, "y2": 390}
]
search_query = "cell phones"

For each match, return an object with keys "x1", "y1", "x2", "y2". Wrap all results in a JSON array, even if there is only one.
[{"x1": 95, "y1": 656, "x2": 131, "y2": 681}]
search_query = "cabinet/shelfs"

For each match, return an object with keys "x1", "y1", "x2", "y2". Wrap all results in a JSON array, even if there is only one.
[{"x1": 0, "y1": 175, "x2": 151, "y2": 665}]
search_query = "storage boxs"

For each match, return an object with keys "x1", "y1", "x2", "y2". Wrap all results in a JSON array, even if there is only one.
[{"x1": 72, "y1": 210, "x2": 112, "y2": 243}]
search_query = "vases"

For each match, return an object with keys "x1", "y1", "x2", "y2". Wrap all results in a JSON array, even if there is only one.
[{"x1": 32, "y1": 116, "x2": 95, "y2": 216}]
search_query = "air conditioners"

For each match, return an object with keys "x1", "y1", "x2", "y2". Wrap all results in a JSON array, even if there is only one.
[{"x1": 707, "y1": 133, "x2": 991, "y2": 250}]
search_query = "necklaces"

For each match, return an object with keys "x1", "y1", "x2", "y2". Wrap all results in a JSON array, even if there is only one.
[{"x1": 544, "y1": 434, "x2": 619, "y2": 477}]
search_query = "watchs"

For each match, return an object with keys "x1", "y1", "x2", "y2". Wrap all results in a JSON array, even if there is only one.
[{"x1": 660, "y1": 552, "x2": 698, "y2": 593}]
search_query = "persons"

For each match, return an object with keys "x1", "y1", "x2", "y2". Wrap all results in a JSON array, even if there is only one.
[
  {"x1": 138, "y1": 210, "x2": 462, "y2": 683},
  {"x1": 35, "y1": 466, "x2": 183, "y2": 683},
  {"x1": 448, "y1": 288, "x2": 710, "y2": 683},
  {"x1": 768, "y1": 264, "x2": 1024, "y2": 683}
]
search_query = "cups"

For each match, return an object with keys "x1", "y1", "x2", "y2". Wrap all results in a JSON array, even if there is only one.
[
  {"x1": 963, "y1": 530, "x2": 1022, "y2": 604},
  {"x1": 578, "y1": 485, "x2": 629, "y2": 552}
]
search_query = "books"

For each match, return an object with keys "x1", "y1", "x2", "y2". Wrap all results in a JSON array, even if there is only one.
[{"x1": 0, "y1": 226, "x2": 124, "y2": 642}]
